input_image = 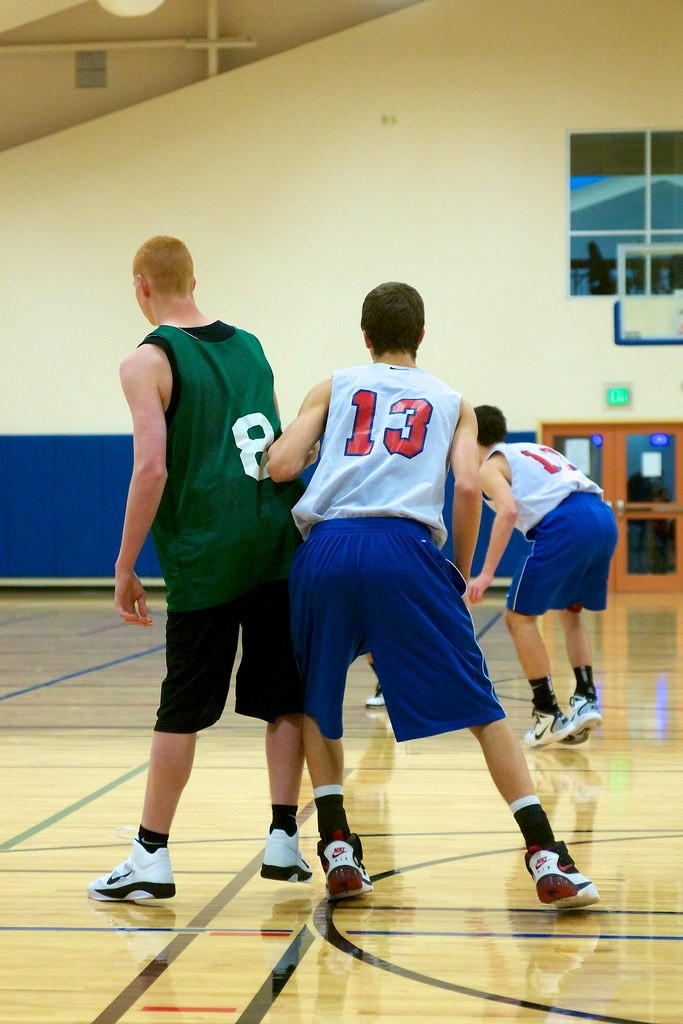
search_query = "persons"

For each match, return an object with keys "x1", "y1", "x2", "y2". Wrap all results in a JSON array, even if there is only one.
[
  {"x1": 93, "y1": 709, "x2": 601, "y2": 1024},
  {"x1": 466, "y1": 405, "x2": 618, "y2": 748},
  {"x1": 362, "y1": 649, "x2": 386, "y2": 707},
  {"x1": 266, "y1": 283, "x2": 602, "y2": 908},
  {"x1": 87, "y1": 235, "x2": 312, "y2": 902}
]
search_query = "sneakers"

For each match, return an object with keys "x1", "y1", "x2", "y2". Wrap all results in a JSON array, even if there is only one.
[
  {"x1": 261, "y1": 822, "x2": 312, "y2": 883},
  {"x1": 366, "y1": 681, "x2": 387, "y2": 709},
  {"x1": 557, "y1": 693, "x2": 602, "y2": 745},
  {"x1": 317, "y1": 829, "x2": 372, "y2": 900},
  {"x1": 524, "y1": 707, "x2": 577, "y2": 750},
  {"x1": 525, "y1": 841, "x2": 599, "y2": 909},
  {"x1": 87, "y1": 827, "x2": 176, "y2": 901}
]
